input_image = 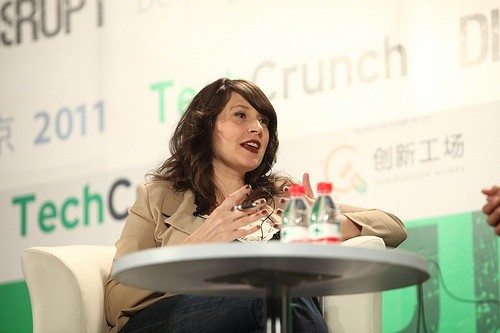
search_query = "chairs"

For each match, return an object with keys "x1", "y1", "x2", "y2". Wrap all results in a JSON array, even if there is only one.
[{"x1": 20, "y1": 235, "x2": 387, "y2": 333}]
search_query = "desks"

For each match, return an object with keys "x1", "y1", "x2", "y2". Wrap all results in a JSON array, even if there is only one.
[{"x1": 112, "y1": 241, "x2": 429, "y2": 333}]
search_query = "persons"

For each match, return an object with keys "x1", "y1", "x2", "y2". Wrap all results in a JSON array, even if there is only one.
[{"x1": 104, "y1": 77, "x2": 408, "y2": 333}]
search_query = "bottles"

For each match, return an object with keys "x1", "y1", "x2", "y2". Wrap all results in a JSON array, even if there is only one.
[
  {"x1": 279, "y1": 184, "x2": 312, "y2": 243},
  {"x1": 308, "y1": 182, "x2": 342, "y2": 243}
]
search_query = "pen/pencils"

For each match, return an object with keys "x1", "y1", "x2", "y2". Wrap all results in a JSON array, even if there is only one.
[{"x1": 234, "y1": 202, "x2": 258, "y2": 210}]
